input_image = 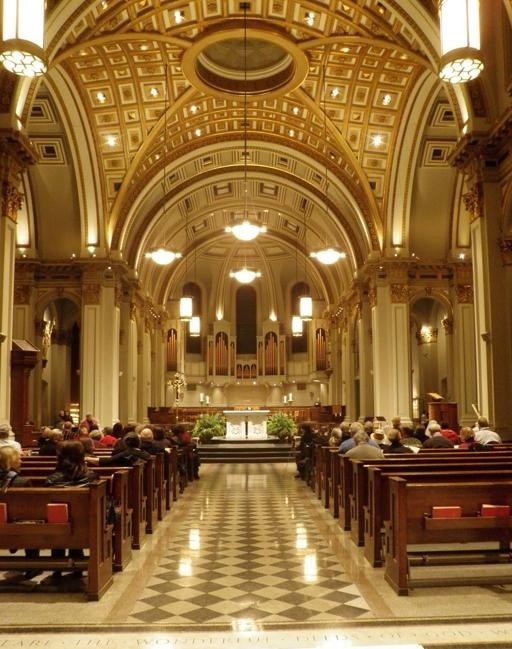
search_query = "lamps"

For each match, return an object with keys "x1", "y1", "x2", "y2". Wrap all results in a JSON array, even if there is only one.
[
  {"x1": 221, "y1": 0, "x2": 272, "y2": 244},
  {"x1": 228, "y1": 248, "x2": 266, "y2": 288},
  {"x1": 0, "y1": 0, "x2": 49, "y2": 77},
  {"x1": 287, "y1": 288, "x2": 324, "y2": 338},
  {"x1": 170, "y1": 292, "x2": 209, "y2": 338},
  {"x1": 437, "y1": 0, "x2": 485, "y2": 84},
  {"x1": 307, "y1": 65, "x2": 346, "y2": 268},
  {"x1": 142, "y1": 59, "x2": 189, "y2": 269}
]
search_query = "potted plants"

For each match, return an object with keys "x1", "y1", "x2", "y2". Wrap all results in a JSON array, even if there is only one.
[
  {"x1": 190, "y1": 412, "x2": 228, "y2": 444},
  {"x1": 268, "y1": 412, "x2": 298, "y2": 442}
]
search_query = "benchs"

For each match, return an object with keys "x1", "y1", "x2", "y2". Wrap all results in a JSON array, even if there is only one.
[
  {"x1": 0, "y1": 440, "x2": 197, "y2": 600},
  {"x1": 294, "y1": 435, "x2": 512, "y2": 595}
]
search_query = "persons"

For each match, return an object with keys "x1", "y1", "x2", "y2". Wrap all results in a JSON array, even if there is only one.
[
  {"x1": 38, "y1": 409, "x2": 200, "y2": 499},
  {"x1": 292, "y1": 410, "x2": 503, "y2": 479},
  {"x1": 0, "y1": 444, "x2": 42, "y2": 579},
  {"x1": 0, "y1": 423, "x2": 22, "y2": 452},
  {"x1": 38, "y1": 440, "x2": 97, "y2": 586}
]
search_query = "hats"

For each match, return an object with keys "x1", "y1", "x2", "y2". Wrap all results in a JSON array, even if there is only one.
[
  {"x1": 0, "y1": 422, "x2": 12, "y2": 433},
  {"x1": 370, "y1": 429, "x2": 387, "y2": 445},
  {"x1": 389, "y1": 429, "x2": 402, "y2": 440}
]
